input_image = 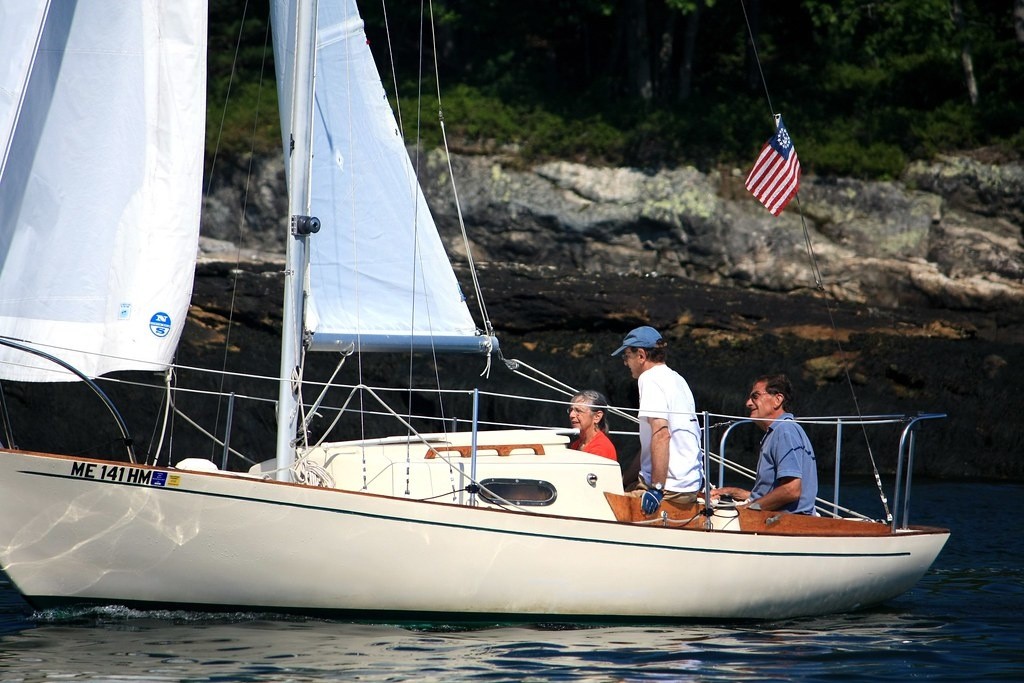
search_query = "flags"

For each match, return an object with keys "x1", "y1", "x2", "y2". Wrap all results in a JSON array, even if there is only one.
[{"x1": 744, "y1": 116, "x2": 802, "y2": 217}]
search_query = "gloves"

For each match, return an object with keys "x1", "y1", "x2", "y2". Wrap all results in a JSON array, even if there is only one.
[{"x1": 640, "y1": 488, "x2": 666, "y2": 517}]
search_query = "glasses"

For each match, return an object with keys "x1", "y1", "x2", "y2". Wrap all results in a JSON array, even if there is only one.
[
  {"x1": 567, "y1": 406, "x2": 594, "y2": 415},
  {"x1": 747, "y1": 390, "x2": 778, "y2": 401},
  {"x1": 622, "y1": 352, "x2": 638, "y2": 362}
]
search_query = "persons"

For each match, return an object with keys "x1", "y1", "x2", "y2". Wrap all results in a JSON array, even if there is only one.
[
  {"x1": 610, "y1": 325, "x2": 703, "y2": 519},
  {"x1": 568, "y1": 389, "x2": 618, "y2": 462},
  {"x1": 710, "y1": 373, "x2": 819, "y2": 516}
]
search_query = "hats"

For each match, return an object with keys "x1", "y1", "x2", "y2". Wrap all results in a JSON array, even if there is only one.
[{"x1": 611, "y1": 326, "x2": 667, "y2": 356}]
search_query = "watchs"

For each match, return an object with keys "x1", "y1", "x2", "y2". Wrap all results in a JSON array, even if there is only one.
[{"x1": 652, "y1": 482, "x2": 664, "y2": 490}]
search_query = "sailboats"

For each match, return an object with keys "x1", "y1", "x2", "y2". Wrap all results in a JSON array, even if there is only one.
[{"x1": 0, "y1": 0, "x2": 951, "y2": 622}]
